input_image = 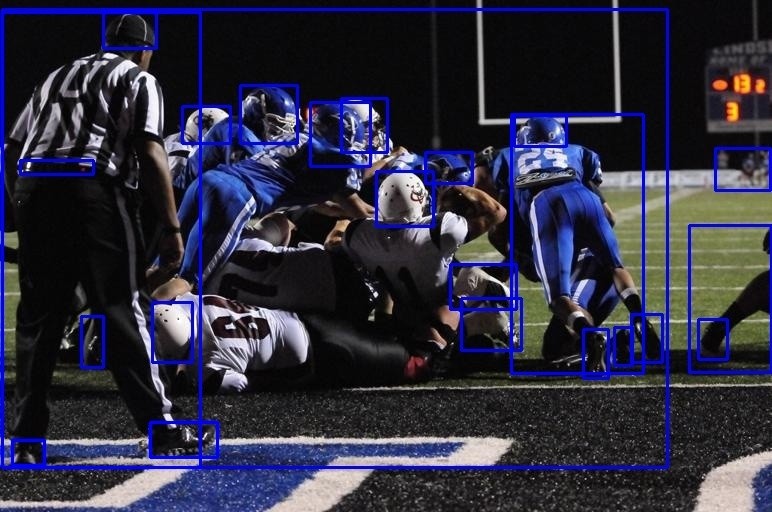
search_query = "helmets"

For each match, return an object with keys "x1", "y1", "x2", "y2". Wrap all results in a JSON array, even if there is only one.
[
  {"x1": 377, "y1": 171, "x2": 434, "y2": 226},
  {"x1": 183, "y1": 106, "x2": 230, "y2": 143},
  {"x1": 153, "y1": 303, "x2": 193, "y2": 362},
  {"x1": 240, "y1": 85, "x2": 298, "y2": 143},
  {"x1": 341, "y1": 99, "x2": 388, "y2": 152},
  {"x1": 311, "y1": 102, "x2": 371, "y2": 167},
  {"x1": 426, "y1": 153, "x2": 472, "y2": 184},
  {"x1": 515, "y1": 116, "x2": 566, "y2": 146}
]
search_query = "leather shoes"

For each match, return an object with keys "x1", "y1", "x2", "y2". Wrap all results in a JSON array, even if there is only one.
[
  {"x1": 12, "y1": 440, "x2": 44, "y2": 465},
  {"x1": 151, "y1": 423, "x2": 217, "y2": 458}
]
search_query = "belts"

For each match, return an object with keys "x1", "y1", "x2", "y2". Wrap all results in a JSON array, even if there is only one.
[{"x1": 20, "y1": 160, "x2": 93, "y2": 174}]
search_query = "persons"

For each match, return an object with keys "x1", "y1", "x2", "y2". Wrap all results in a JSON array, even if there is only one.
[
  {"x1": 715, "y1": 148, "x2": 770, "y2": 191},
  {"x1": 459, "y1": 132, "x2": 637, "y2": 376},
  {"x1": 1, "y1": 12, "x2": 215, "y2": 466},
  {"x1": 689, "y1": 225, "x2": 772, "y2": 373},
  {"x1": 59, "y1": 85, "x2": 524, "y2": 395}
]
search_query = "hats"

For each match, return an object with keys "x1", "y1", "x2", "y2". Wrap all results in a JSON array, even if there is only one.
[{"x1": 104, "y1": 13, "x2": 157, "y2": 48}]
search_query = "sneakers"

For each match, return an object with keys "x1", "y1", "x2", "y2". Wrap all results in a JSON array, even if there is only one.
[
  {"x1": 584, "y1": 329, "x2": 608, "y2": 379},
  {"x1": 461, "y1": 299, "x2": 521, "y2": 351},
  {"x1": 632, "y1": 314, "x2": 663, "y2": 362},
  {"x1": 614, "y1": 328, "x2": 633, "y2": 366},
  {"x1": 699, "y1": 319, "x2": 728, "y2": 360},
  {"x1": 81, "y1": 317, "x2": 104, "y2": 368},
  {"x1": 451, "y1": 264, "x2": 517, "y2": 309}
]
